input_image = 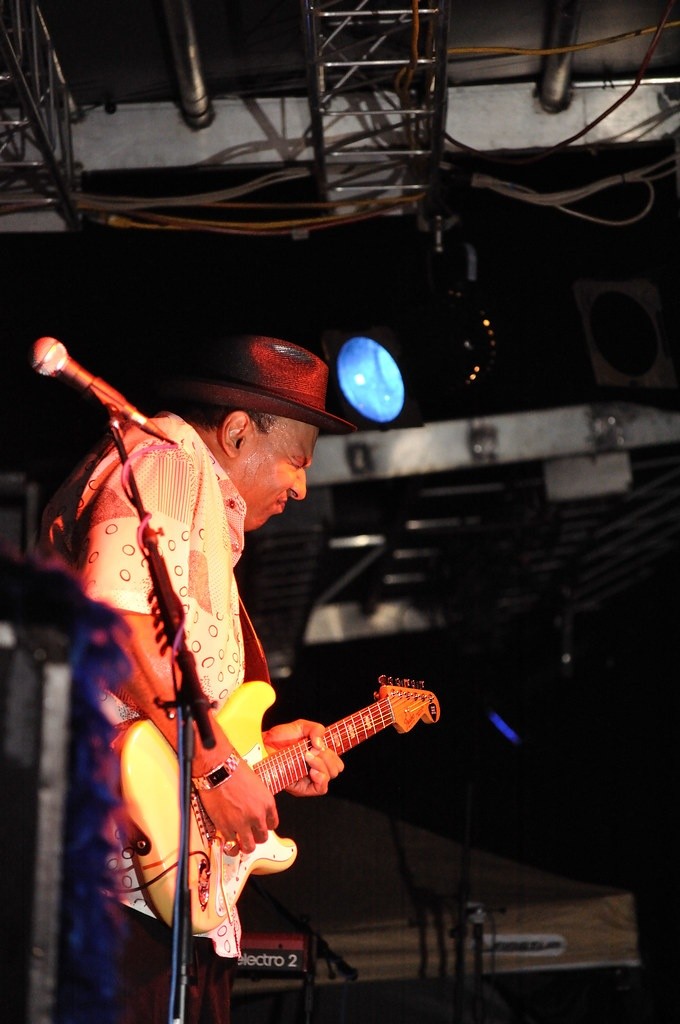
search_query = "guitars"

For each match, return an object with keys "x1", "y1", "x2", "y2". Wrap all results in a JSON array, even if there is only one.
[{"x1": 104, "y1": 672, "x2": 442, "y2": 936}]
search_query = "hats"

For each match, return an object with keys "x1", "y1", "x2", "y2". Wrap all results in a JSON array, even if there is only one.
[{"x1": 151, "y1": 334, "x2": 358, "y2": 435}]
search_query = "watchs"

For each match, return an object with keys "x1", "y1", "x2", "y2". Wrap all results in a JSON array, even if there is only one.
[{"x1": 192, "y1": 746, "x2": 240, "y2": 790}]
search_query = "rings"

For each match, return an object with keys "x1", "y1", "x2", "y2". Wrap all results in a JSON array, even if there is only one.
[{"x1": 221, "y1": 840, "x2": 236, "y2": 852}]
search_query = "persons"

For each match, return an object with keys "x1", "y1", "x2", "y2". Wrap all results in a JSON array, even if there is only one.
[{"x1": 28, "y1": 337, "x2": 357, "y2": 1024}]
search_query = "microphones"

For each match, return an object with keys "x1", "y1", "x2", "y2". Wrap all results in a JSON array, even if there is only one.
[{"x1": 28, "y1": 336, "x2": 168, "y2": 443}]
